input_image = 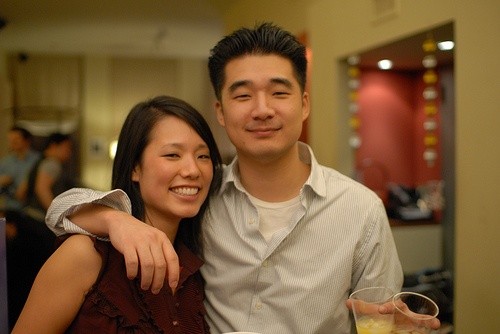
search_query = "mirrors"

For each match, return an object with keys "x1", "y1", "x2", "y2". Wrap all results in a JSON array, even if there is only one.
[{"x1": 337, "y1": 17, "x2": 454, "y2": 230}]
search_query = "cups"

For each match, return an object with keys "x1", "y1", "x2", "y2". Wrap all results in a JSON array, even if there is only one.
[
  {"x1": 349, "y1": 287, "x2": 394, "y2": 334},
  {"x1": 392, "y1": 292, "x2": 439, "y2": 334}
]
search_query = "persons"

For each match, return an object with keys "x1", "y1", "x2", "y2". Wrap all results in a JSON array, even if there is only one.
[
  {"x1": 10, "y1": 95, "x2": 224, "y2": 334},
  {"x1": 45, "y1": 21, "x2": 441, "y2": 334},
  {"x1": 0, "y1": 126, "x2": 86, "y2": 289}
]
